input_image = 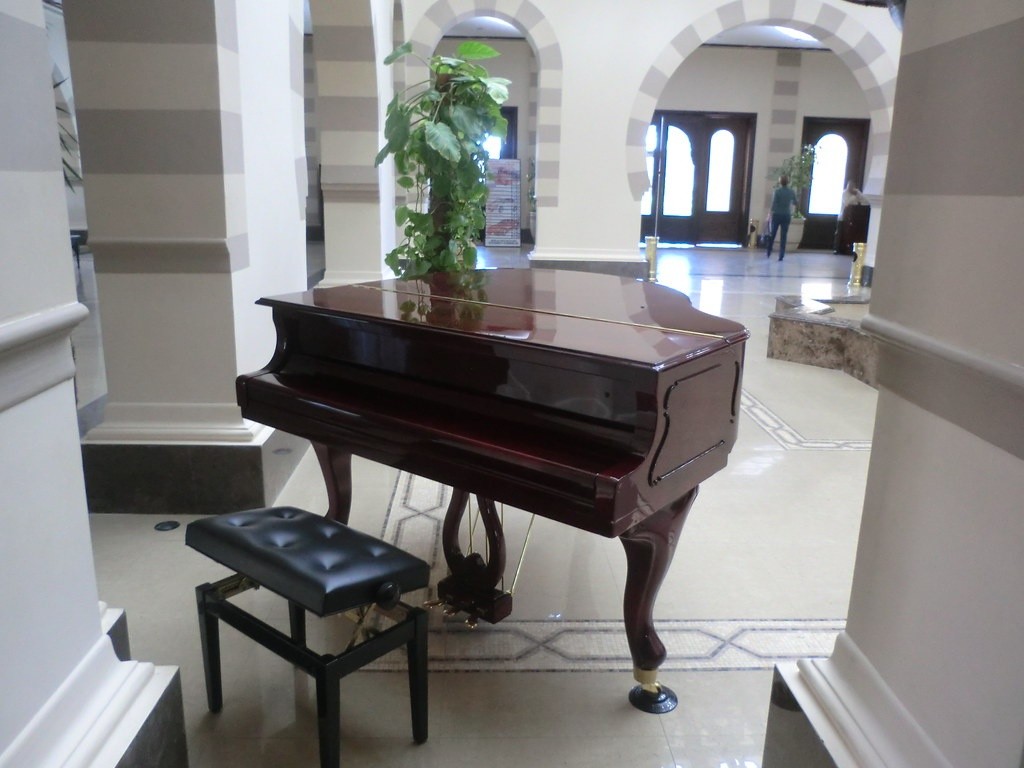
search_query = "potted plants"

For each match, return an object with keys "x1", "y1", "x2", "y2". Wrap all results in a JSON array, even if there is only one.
[{"x1": 767, "y1": 143, "x2": 819, "y2": 250}]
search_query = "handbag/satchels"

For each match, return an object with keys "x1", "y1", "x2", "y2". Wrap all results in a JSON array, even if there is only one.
[{"x1": 762, "y1": 222, "x2": 771, "y2": 243}]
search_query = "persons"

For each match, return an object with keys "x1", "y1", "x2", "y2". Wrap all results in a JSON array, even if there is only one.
[
  {"x1": 833, "y1": 180, "x2": 870, "y2": 254},
  {"x1": 767, "y1": 176, "x2": 800, "y2": 261}
]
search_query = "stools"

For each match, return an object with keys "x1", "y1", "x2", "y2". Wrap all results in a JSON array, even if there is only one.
[{"x1": 185, "y1": 506, "x2": 429, "y2": 768}]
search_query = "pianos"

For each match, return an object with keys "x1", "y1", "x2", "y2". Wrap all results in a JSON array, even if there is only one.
[{"x1": 233, "y1": 265, "x2": 752, "y2": 716}]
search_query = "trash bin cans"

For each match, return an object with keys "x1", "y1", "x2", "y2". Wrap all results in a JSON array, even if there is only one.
[
  {"x1": 645, "y1": 236, "x2": 658, "y2": 281},
  {"x1": 747, "y1": 218, "x2": 759, "y2": 248},
  {"x1": 848, "y1": 242, "x2": 866, "y2": 286}
]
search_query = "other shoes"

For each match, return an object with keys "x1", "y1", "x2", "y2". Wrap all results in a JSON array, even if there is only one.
[
  {"x1": 766, "y1": 251, "x2": 771, "y2": 256},
  {"x1": 779, "y1": 258, "x2": 783, "y2": 261},
  {"x1": 833, "y1": 250, "x2": 839, "y2": 255}
]
